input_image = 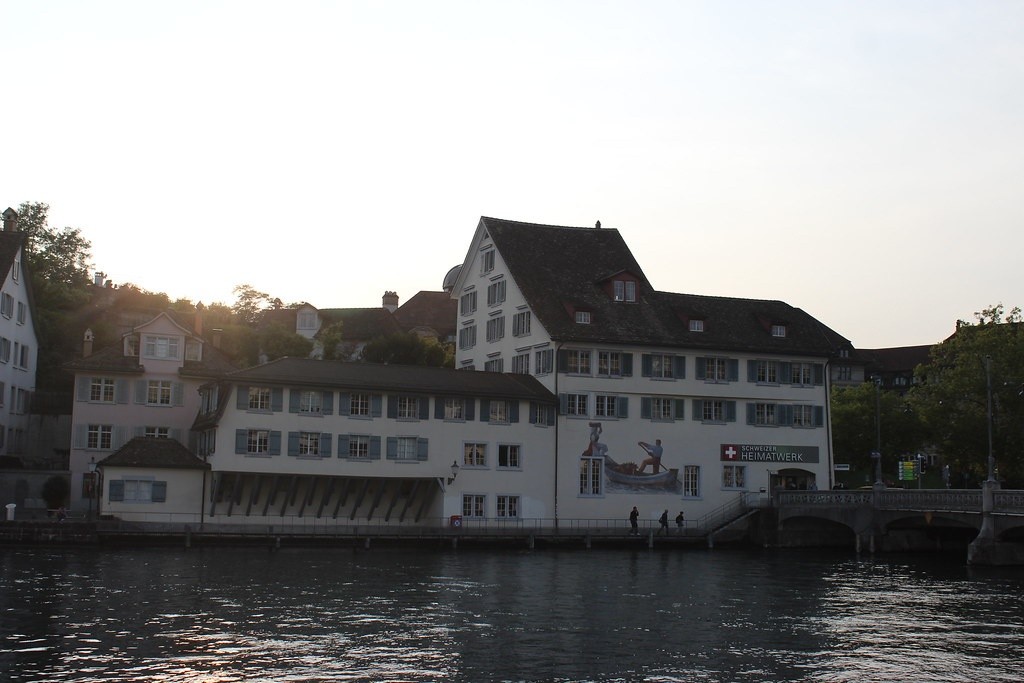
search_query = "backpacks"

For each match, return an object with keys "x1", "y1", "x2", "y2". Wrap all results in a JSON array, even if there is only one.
[{"x1": 676, "y1": 515, "x2": 681, "y2": 523}]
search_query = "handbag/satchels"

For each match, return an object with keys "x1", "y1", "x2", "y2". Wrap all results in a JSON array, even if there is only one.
[{"x1": 659, "y1": 517, "x2": 662, "y2": 522}]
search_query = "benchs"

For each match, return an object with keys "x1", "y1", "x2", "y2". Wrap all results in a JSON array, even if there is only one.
[{"x1": 45, "y1": 510, "x2": 86, "y2": 521}]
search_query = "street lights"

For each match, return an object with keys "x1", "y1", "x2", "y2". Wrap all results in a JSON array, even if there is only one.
[
  {"x1": 870, "y1": 375, "x2": 881, "y2": 451},
  {"x1": 986, "y1": 354, "x2": 994, "y2": 479},
  {"x1": 86, "y1": 457, "x2": 98, "y2": 521}
]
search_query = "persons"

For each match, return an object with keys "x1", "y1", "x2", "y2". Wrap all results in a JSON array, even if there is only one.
[
  {"x1": 810, "y1": 480, "x2": 818, "y2": 490},
  {"x1": 675, "y1": 511, "x2": 685, "y2": 536},
  {"x1": 629, "y1": 507, "x2": 641, "y2": 536},
  {"x1": 656, "y1": 509, "x2": 670, "y2": 536},
  {"x1": 57, "y1": 505, "x2": 67, "y2": 521}
]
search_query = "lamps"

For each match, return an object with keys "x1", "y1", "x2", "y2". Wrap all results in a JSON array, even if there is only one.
[{"x1": 447, "y1": 460, "x2": 459, "y2": 485}]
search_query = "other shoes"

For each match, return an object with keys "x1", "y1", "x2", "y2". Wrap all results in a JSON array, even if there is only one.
[
  {"x1": 630, "y1": 533, "x2": 634, "y2": 535},
  {"x1": 637, "y1": 533, "x2": 640, "y2": 535}
]
